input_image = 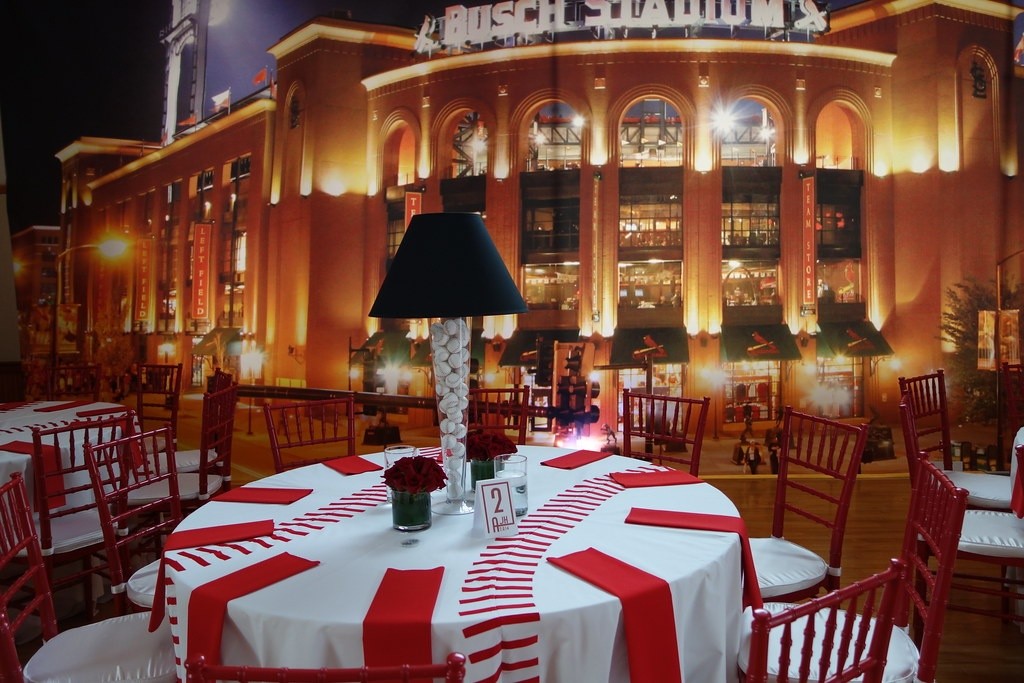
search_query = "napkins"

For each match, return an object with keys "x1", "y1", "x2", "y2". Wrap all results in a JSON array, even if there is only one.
[
  {"x1": 419, "y1": 446, "x2": 453, "y2": 463},
  {"x1": 1009, "y1": 446, "x2": 1023, "y2": 520},
  {"x1": 208, "y1": 485, "x2": 314, "y2": 505},
  {"x1": 363, "y1": 565, "x2": 444, "y2": 683},
  {"x1": 539, "y1": 448, "x2": 612, "y2": 470},
  {"x1": 186, "y1": 552, "x2": 321, "y2": 669},
  {"x1": 1, "y1": 400, "x2": 28, "y2": 412},
  {"x1": 74, "y1": 405, "x2": 136, "y2": 419},
  {"x1": 0, "y1": 440, "x2": 68, "y2": 513},
  {"x1": 625, "y1": 505, "x2": 762, "y2": 613},
  {"x1": 33, "y1": 398, "x2": 96, "y2": 414},
  {"x1": 606, "y1": 469, "x2": 705, "y2": 489},
  {"x1": 322, "y1": 454, "x2": 384, "y2": 476},
  {"x1": 148, "y1": 519, "x2": 274, "y2": 631},
  {"x1": 545, "y1": 546, "x2": 682, "y2": 683},
  {"x1": 67, "y1": 417, "x2": 144, "y2": 472}
]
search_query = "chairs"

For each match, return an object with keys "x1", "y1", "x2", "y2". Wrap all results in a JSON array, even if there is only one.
[
  {"x1": 0, "y1": 354, "x2": 374, "y2": 666},
  {"x1": 166, "y1": 439, "x2": 753, "y2": 683},
  {"x1": 461, "y1": 382, "x2": 534, "y2": 445},
  {"x1": 896, "y1": 365, "x2": 1017, "y2": 614},
  {"x1": 621, "y1": 387, "x2": 711, "y2": 479},
  {"x1": 747, "y1": 404, "x2": 870, "y2": 606},
  {"x1": 740, "y1": 559, "x2": 922, "y2": 683},
  {"x1": 897, "y1": 394, "x2": 1023, "y2": 657}
]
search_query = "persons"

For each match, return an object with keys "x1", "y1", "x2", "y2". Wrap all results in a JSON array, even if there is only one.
[
  {"x1": 775, "y1": 404, "x2": 784, "y2": 429},
  {"x1": 743, "y1": 402, "x2": 753, "y2": 429},
  {"x1": 768, "y1": 443, "x2": 780, "y2": 475},
  {"x1": 127, "y1": 361, "x2": 154, "y2": 392},
  {"x1": 868, "y1": 405, "x2": 885, "y2": 427},
  {"x1": 744, "y1": 441, "x2": 761, "y2": 474}
]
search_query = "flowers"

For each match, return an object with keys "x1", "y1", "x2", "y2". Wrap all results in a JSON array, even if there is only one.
[
  {"x1": 464, "y1": 426, "x2": 520, "y2": 462},
  {"x1": 380, "y1": 457, "x2": 449, "y2": 501}
]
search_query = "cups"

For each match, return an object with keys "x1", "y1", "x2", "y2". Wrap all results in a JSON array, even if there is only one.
[
  {"x1": 384, "y1": 445, "x2": 418, "y2": 503},
  {"x1": 493, "y1": 453, "x2": 528, "y2": 516}
]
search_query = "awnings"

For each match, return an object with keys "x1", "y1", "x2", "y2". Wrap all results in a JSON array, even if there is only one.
[
  {"x1": 611, "y1": 326, "x2": 691, "y2": 366},
  {"x1": 497, "y1": 327, "x2": 581, "y2": 369},
  {"x1": 409, "y1": 327, "x2": 485, "y2": 366},
  {"x1": 190, "y1": 327, "x2": 243, "y2": 355},
  {"x1": 720, "y1": 323, "x2": 803, "y2": 362},
  {"x1": 349, "y1": 330, "x2": 411, "y2": 368},
  {"x1": 816, "y1": 319, "x2": 895, "y2": 359}
]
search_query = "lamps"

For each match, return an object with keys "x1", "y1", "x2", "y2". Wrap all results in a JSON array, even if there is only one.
[
  {"x1": 367, "y1": 211, "x2": 529, "y2": 516},
  {"x1": 798, "y1": 168, "x2": 815, "y2": 179},
  {"x1": 799, "y1": 303, "x2": 817, "y2": 316},
  {"x1": 970, "y1": 61, "x2": 987, "y2": 97}
]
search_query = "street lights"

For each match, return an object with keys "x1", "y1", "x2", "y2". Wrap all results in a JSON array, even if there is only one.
[{"x1": 52, "y1": 241, "x2": 125, "y2": 400}]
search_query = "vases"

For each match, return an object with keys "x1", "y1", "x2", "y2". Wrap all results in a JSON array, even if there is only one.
[
  {"x1": 471, "y1": 459, "x2": 494, "y2": 492},
  {"x1": 390, "y1": 488, "x2": 431, "y2": 530}
]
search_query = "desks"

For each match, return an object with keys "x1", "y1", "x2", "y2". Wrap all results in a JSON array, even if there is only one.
[{"x1": 0, "y1": 396, "x2": 152, "y2": 594}]
